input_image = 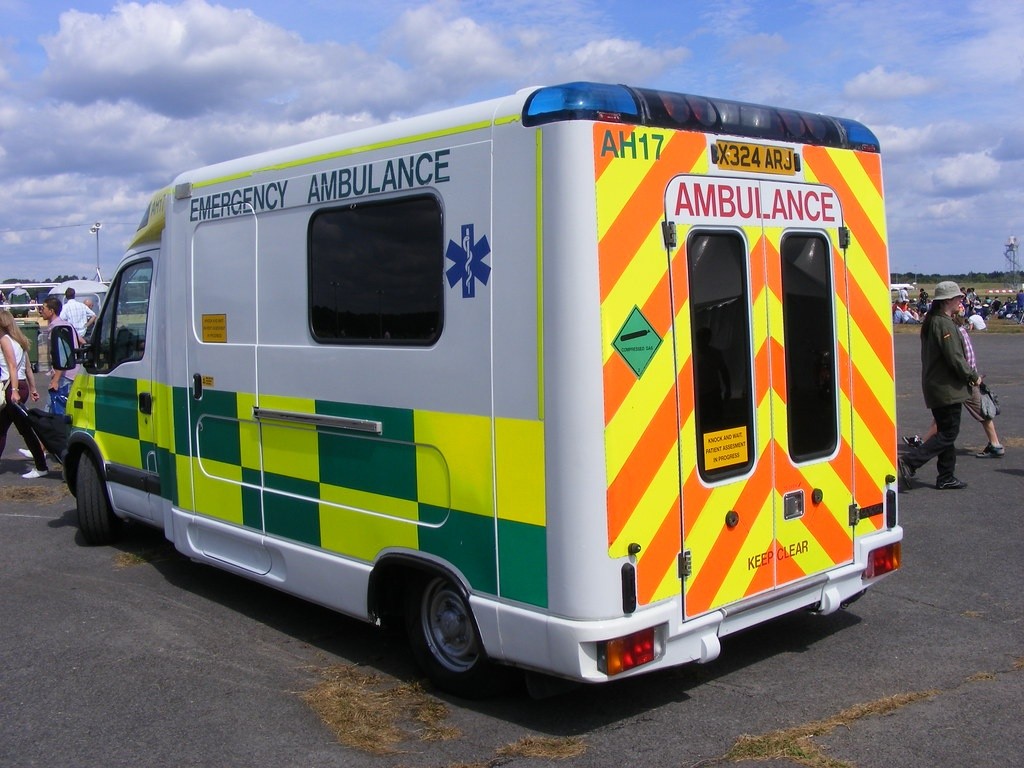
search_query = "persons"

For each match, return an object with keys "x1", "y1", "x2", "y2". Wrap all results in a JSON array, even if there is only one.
[
  {"x1": 57, "y1": 287, "x2": 95, "y2": 351},
  {"x1": 897, "y1": 281, "x2": 982, "y2": 490},
  {"x1": 131, "y1": 340, "x2": 145, "y2": 359},
  {"x1": 892, "y1": 287, "x2": 1024, "y2": 331},
  {"x1": 901, "y1": 302, "x2": 1005, "y2": 459},
  {"x1": 0, "y1": 281, "x2": 32, "y2": 318},
  {"x1": 0, "y1": 310, "x2": 49, "y2": 479},
  {"x1": 17, "y1": 296, "x2": 87, "y2": 461}
]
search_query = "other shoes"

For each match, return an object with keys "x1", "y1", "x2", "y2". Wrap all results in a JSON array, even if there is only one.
[
  {"x1": 898, "y1": 458, "x2": 914, "y2": 489},
  {"x1": 936, "y1": 475, "x2": 968, "y2": 490}
]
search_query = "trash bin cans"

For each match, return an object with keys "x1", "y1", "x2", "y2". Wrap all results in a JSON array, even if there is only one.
[{"x1": 14, "y1": 320, "x2": 41, "y2": 373}]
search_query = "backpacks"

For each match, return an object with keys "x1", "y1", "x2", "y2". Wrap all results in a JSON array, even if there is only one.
[{"x1": 48, "y1": 383, "x2": 69, "y2": 415}]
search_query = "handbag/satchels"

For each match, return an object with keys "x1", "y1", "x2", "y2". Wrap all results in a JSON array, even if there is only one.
[
  {"x1": 0, "y1": 382, "x2": 7, "y2": 410},
  {"x1": 981, "y1": 382, "x2": 1001, "y2": 418}
]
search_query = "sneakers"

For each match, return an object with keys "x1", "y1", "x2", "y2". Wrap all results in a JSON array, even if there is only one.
[
  {"x1": 19, "y1": 448, "x2": 47, "y2": 459},
  {"x1": 22, "y1": 468, "x2": 48, "y2": 479},
  {"x1": 902, "y1": 435, "x2": 924, "y2": 449},
  {"x1": 976, "y1": 442, "x2": 1005, "y2": 457}
]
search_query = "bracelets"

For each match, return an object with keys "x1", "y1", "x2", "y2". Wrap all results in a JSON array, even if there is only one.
[{"x1": 10, "y1": 388, "x2": 19, "y2": 391}]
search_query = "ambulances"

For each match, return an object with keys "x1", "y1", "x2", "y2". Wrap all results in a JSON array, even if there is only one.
[{"x1": 50, "y1": 80, "x2": 904, "y2": 700}]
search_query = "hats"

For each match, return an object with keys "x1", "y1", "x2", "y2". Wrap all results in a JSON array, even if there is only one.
[{"x1": 931, "y1": 281, "x2": 965, "y2": 300}]
